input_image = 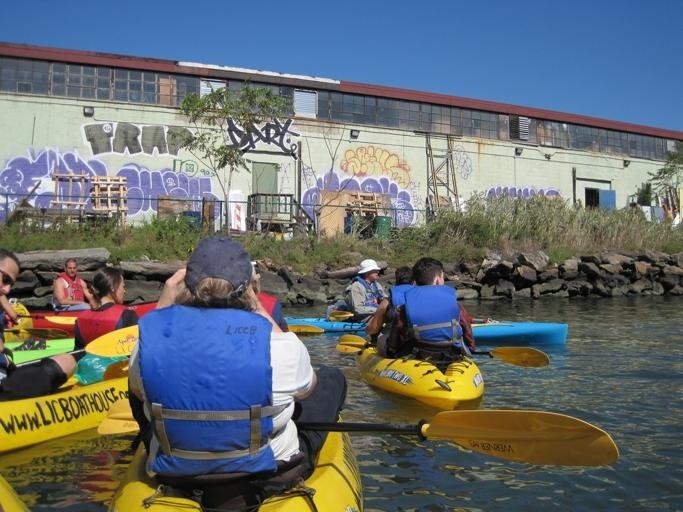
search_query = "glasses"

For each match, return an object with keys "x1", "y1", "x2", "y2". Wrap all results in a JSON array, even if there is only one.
[{"x1": 0, "y1": 270, "x2": 14, "y2": 287}]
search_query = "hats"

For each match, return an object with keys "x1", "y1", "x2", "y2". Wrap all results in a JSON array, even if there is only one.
[
  {"x1": 185, "y1": 236, "x2": 252, "y2": 299},
  {"x1": 358, "y1": 259, "x2": 381, "y2": 274}
]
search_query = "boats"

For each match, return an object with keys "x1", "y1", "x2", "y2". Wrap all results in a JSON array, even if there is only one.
[
  {"x1": 107, "y1": 407, "x2": 364, "y2": 511},
  {"x1": 357, "y1": 341, "x2": 486, "y2": 413},
  {"x1": 285, "y1": 310, "x2": 570, "y2": 351}
]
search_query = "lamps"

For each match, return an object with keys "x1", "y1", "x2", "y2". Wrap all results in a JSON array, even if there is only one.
[
  {"x1": 348, "y1": 128, "x2": 359, "y2": 139},
  {"x1": 622, "y1": 159, "x2": 630, "y2": 167},
  {"x1": 513, "y1": 147, "x2": 523, "y2": 155},
  {"x1": 82, "y1": 105, "x2": 94, "y2": 116}
]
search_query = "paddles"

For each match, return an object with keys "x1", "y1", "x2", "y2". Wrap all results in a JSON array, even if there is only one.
[
  {"x1": 335, "y1": 334, "x2": 550, "y2": 366},
  {"x1": 17, "y1": 315, "x2": 77, "y2": 325},
  {"x1": 288, "y1": 324, "x2": 324, "y2": 333},
  {"x1": 4, "y1": 324, "x2": 139, "y2": 380},
  {"x1": 98, "y1": 399, "x2": 620, "y2": 467},
  {"x1": 328, "y1": 310, "x2": 374, "y2": 322}
]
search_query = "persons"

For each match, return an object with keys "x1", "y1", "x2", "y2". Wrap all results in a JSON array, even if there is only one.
[
  {"x1": 378, "y1": 257, "x2": 475, "y2": 372},
  {"x1": 51, "y1": 259, "x2": 98, "y2": 312},
  {"x1": 346, "y1": 259, "x2": 388, "y2": 323},
  {"x1": 72, "y1": 267, "x2": 138, "y2": 363},
  {"x1": 128, "y1": 234, "x2": 346, "y2": 512},
  {"x1": 0, "y1": 248, "x2": 77, "y2": 402},
  {"x1": 365, "y1": 267, "x2": 416, "y2": 359},
  {"x1": 249, "y1": 261, "x2": 288, "y2": 335}
]
